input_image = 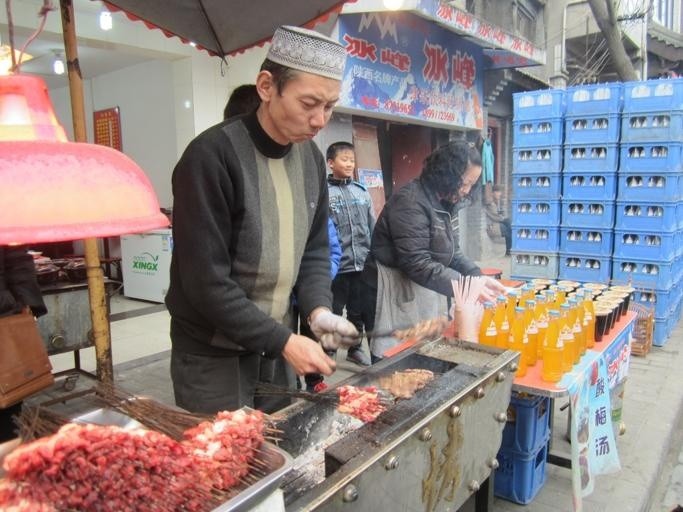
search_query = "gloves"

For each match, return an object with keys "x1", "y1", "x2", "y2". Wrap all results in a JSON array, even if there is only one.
[{"x1": 310, "y1": 310, "x2": 360, "y2": 351}]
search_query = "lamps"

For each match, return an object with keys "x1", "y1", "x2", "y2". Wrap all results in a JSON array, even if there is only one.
[
  {"x1": 52, "y1": 48, "x2": 64, "y2": 74},
  {"x1": 100, "y1": 12, "x2": 113, "y2": 30}
]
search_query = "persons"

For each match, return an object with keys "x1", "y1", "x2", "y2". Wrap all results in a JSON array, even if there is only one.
[
  {"x1": 165, "y1": 25, "x2": 361, "y2": 415},
  {"x1": 290, "y1": 217, "x2": 342, "y2": 396},
  {"x1": 0, "y1": 247, "x2": 47, "y2": 444},
  {"x1": 486, "y1": 185, "x2": 512, "y2": 256},
  {"x1": 361, "y1": 140, "x2": 506, "y2": 365},
  {"x1": 224, "y1": 86, "x2": 262, "y2": 120},
  {"x1": 325, "y1": 143, "x2": 377, "y2": 372}
]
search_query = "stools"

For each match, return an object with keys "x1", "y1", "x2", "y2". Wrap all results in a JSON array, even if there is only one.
[
  {"x1": 99, "y1": 255, "x2": 124, "y2": 295},
  {"x1": 480, "y1": 268, "x2": 503, "y2": 279}
]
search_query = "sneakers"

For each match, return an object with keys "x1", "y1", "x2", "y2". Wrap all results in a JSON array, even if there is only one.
[
  {"x1": 326, "y1": 351, "x2": 336, "y2": 360},
  {"x1": 306, "y1": 381, "x2": 329, "y2": 392},
  {"x1": 346, "y1": 349, "x2": 371, "y2": 368}
]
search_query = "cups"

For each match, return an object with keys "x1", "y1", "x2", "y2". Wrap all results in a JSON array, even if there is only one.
[
  {"x1": 525, "y1": 278, "x2": 606, "y2": 297},
  {"x1": 595, "y1": 285, "x2": 636, "y2": 342}
]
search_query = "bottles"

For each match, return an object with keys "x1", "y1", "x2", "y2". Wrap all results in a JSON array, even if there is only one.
[
  {"x1": 584, "y1": 289, "x2": 595, "y2": 349},
  {"x1": 480, "y1": 302, "x2": 496, "y2": 346},
  {"x1": 508, "y1": 307, "x2": 528, "y2": 377},
  {"x1": 568, "y1": 299, "x2": 580, "y2": 364},
  {"x1": 545, "y1": 290, "x2": 558, "y2": 310},
  {"x1": 509, "y1": 291, "x2": 518, "y2": 311},
  {"x1": 496, "y1": 296, "x2": 509, "y2": 348},
  {"x1": 555, "y1": 286, "x2": 567, "y2": 309},
  {"x1": 542, "y1": 311, "x2": 562, "y2": 382},
  {"x1": 527, "y1": 283, "x2": 536, "y2": 300},
  {"x1": 524, "y1": 300, "x2": 538, "y2": 366},
  {"x1": 560, "y1": 304, "x2": 573, "y2": 373},
  {"x1": 536, "y1": 295, "x2": 547, "y2": 359},
  {"x1": 519, "y1": 287, "x2": 528, "y2": 306},
  {"x1": 576, "y1": 294, "x2": 587, "y2": 355}
]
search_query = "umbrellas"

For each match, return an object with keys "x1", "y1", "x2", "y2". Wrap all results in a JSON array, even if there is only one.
[{"x1": 61, "y1": 0, "x2": 358, "y2": 380}]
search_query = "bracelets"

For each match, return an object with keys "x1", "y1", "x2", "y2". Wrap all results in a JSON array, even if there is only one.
[{"x1": 307, "y1": 306, "x2": 329, "y2": 327}]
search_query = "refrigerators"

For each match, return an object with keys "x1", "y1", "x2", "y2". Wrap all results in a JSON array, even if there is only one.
[{"x1": 119, "y1": 228, "x2": 174, "y2": 304}]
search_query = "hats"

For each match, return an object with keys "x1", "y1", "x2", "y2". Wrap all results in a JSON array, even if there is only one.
[{"x1": 266, "y1": 25, "x2": 346, "y2": 81}]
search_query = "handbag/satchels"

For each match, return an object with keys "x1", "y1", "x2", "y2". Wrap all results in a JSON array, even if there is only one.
[{"x1": 1, "y1": 307, "x2": 56, "y2": 411}]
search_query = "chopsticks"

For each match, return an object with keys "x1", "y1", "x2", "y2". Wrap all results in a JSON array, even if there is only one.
[{"x1": 451, "y1": 273, "x2": 489, "y2": 304}]
